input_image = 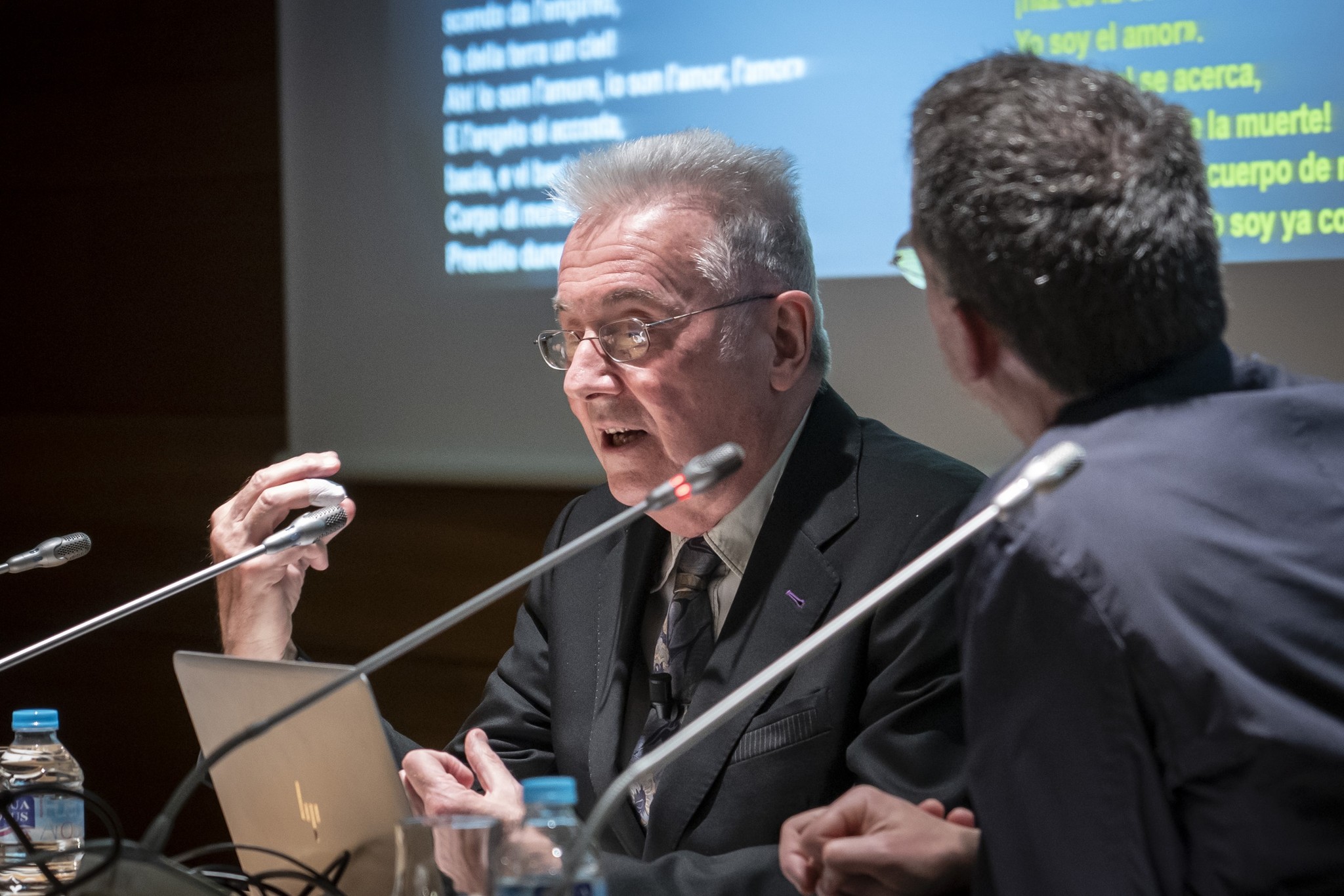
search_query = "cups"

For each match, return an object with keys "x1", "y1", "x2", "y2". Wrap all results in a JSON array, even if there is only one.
[{"x1": 390, "y1": 816, "x2": 498, "y2": 895}]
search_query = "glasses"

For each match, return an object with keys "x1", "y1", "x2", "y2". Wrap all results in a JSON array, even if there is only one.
[
  {"x1": 532, "y1": 291, "x2": 777, "y2": 371},
  {"x1": 891, "y1": 232, "x2": 935, "y2": 289}
]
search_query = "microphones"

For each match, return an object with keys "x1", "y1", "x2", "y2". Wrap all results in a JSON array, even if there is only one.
[
  {"x1": 0, "y1": 532, "x2": 92, "y2": 574},
  {"x1": 73, "y1": 443, "x2": 744, "y2": 896},
  {"x1": 557, "y1": 439, "x2": 1091, "y2": 890},
  {"x1": 0, "y1": 503, "x2": 350, "y2": 672}
]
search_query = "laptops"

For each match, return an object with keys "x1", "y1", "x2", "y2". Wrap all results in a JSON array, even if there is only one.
[{"x1": 173, "y1": 649, "x2": 416, "y2": 896}]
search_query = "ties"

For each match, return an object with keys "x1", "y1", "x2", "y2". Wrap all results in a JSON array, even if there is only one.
[{"x1": 665, "y1": 539, "x2": 723, "y2": 722}]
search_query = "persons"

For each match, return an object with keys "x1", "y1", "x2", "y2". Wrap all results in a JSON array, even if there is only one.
[
  {"x1": 208, "y1": 134, "x2": 988, "y2": 896},
  {"x1": 778, "y1": 50, "x2": 1343, "y2": 895}
]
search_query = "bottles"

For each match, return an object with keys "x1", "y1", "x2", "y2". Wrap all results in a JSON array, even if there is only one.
[
  {"x1": 1, "y1": 709, "x2": 85, "y2": 896},
  {"x1": 486, "y1": 777, "x2": 609, "y2": 896}
]
